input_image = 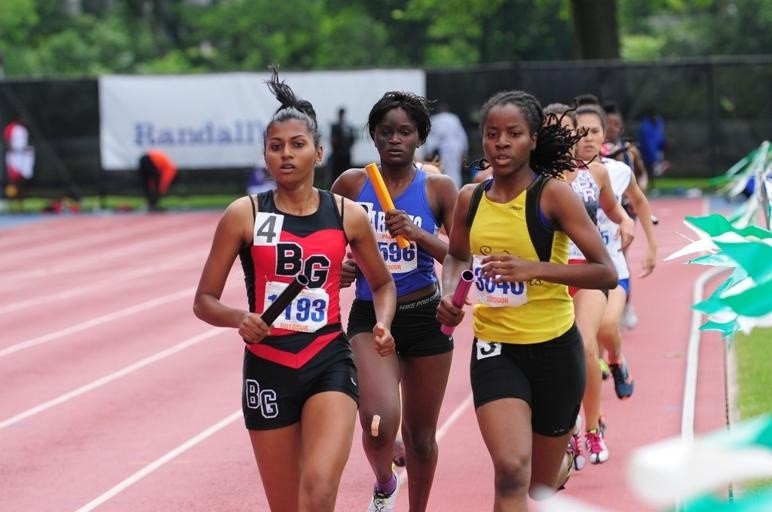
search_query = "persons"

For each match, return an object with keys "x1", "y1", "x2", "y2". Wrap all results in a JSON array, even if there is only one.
[
  {"x1": 392, "y1": 162, "x2": 445, "y2": 467},
  {"x1": 423, "y1": 103, "x2": 469, "y2": 187},
  {"x1": 193, "y1": 63, "x2": 398, "y2": 512},
  {"x1": 329, "y1": 91, "x2": 459, "y2": 512},
  {"x1": 140, "y1": 151, "x2": 181, "y2": 213},
  {"x1": 435, "y1": 91, "x2": 619, "y2": 512},
  {"x1": 327, "y1": 109, "x2": 353, "y2": 183}
]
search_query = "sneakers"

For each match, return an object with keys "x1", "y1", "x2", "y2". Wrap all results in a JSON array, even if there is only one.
[
  {"x1": 586, "y1": 417, "x2": 610, "y2": 466},
  {"x1": 571, "y1": 414, "x2": 586, "y2": 471},
  {"x1": 392, "y1": 437, "x2": 406, "y2": 467},
  {"x1": 608, "y1": 352, "x2": 636, "y2": 399},
  {"x1": 624, "y1": 304, "x2": 637, "y2": 330},
  {"x1": 557, "y1": 434, "x2": 576, "y2": 492},
  {"x1": 599, "y1": 357, "x2": 609, "y2": 380},
  {"x1": 366, "y1": 469, "x2": 402, "y2": 512}
]
